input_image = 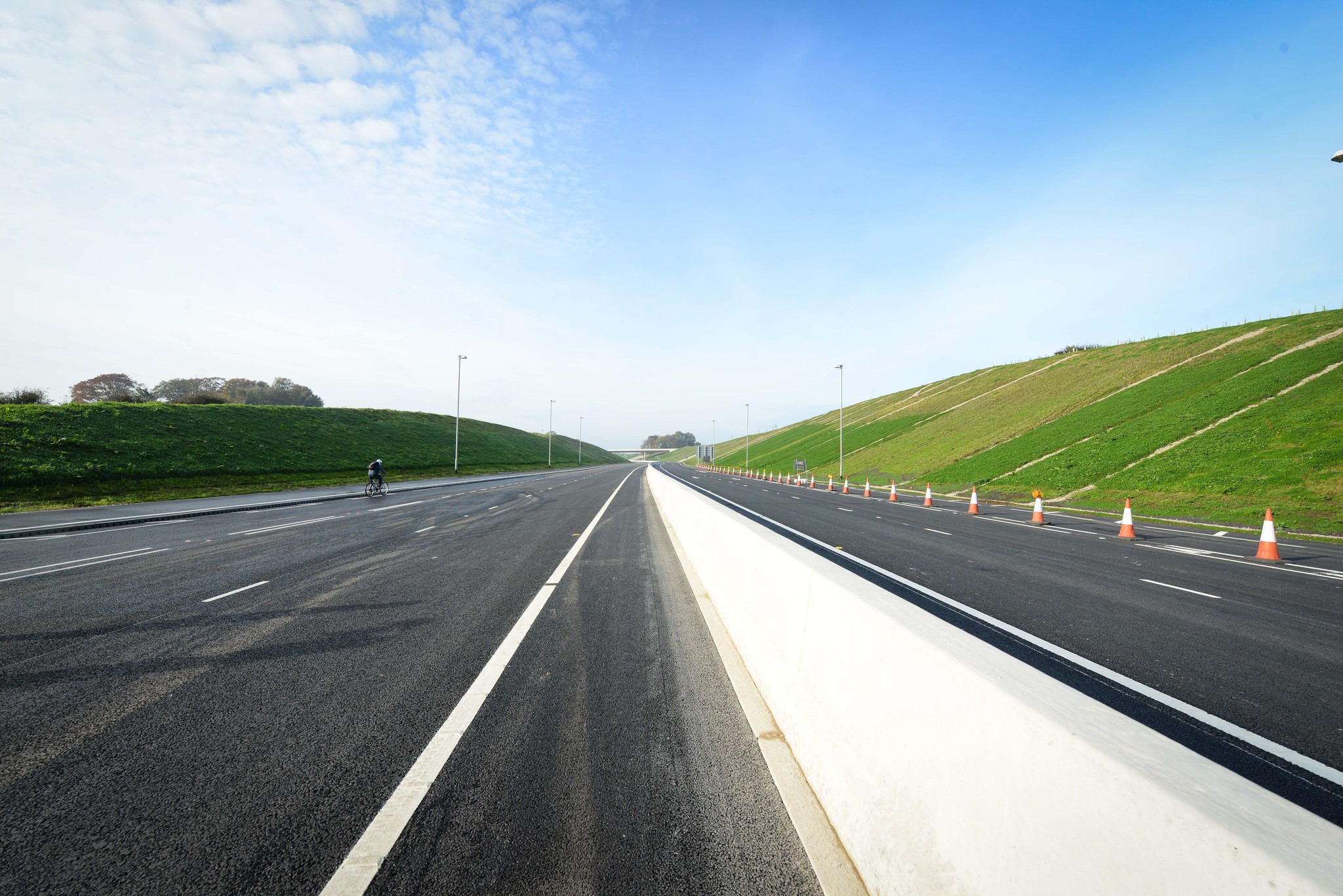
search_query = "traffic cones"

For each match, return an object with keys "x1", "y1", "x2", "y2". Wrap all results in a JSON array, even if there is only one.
[{"x1": 696, "y1": 464, "x2": 1296, "y2": 564}]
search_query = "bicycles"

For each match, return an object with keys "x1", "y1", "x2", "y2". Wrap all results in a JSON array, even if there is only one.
[{"x1": 365, "y1": 474, "x2": 389, "y2": 496}]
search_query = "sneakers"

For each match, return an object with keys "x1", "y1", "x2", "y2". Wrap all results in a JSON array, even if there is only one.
[
  {"x1": 369, "y1": 488, "x2": 371, "y2": 492},
  {"x1": 378, "y1": 486, "x2": 383, "y2": 489}
]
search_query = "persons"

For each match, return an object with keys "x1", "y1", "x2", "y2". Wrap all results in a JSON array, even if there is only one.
[{"x1": 367, "y1": 459, "x2": 388, "y2": 492}]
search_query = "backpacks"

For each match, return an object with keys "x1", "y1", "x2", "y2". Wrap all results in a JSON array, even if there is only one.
[{"x1": 367, "y1": 461, "x2": 379, "y2": 470}]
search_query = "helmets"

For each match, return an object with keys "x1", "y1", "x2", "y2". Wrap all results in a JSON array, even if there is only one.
[{"x1": 376, "y1": 459, "x2": 382, "y2": 465}]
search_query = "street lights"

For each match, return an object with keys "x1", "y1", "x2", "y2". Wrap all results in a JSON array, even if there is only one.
[
  {"x1": 834, "y1": 365, "x2": 843, "y2": 479},
  {"x1": 548, "y1": 400, "x2": 555, "y2": 467},
  {"x1": 745, "y1": 404, "x2": 749, "y2": 469},
  {"x1": 711, "y1": 420, "x2": 714, "y2": 466},
  {"x1": 455, "y1": 355, "x2": 467, "y2": 473},
  {"x1": 578, "y1": 417, "x2": 584, "y2": 465}
]
